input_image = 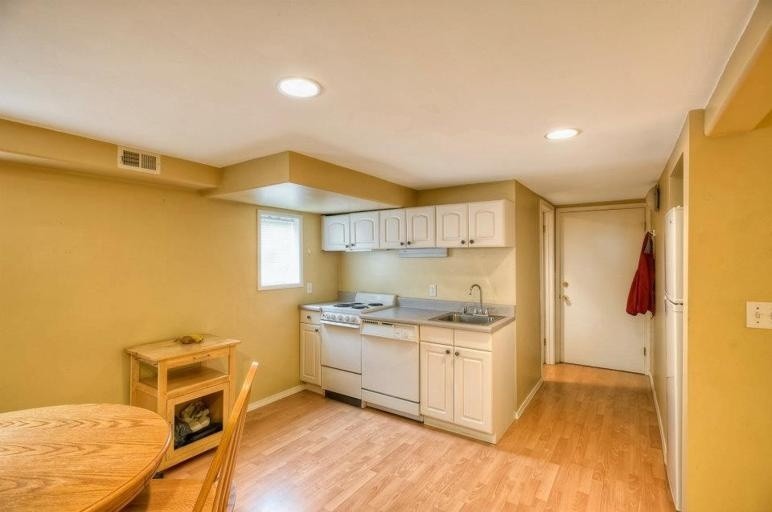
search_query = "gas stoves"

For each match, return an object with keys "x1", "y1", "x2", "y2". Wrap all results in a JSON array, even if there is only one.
[{"x1": 335, "y1": 291, "x2": 388, "y2": 315}]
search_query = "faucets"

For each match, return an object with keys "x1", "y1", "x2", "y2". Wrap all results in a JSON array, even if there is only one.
[{"x1": 467, "y1": 283, "x2": 483, "y2": 315}]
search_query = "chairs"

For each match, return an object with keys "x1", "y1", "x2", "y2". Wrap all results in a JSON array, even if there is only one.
[{"x1": 120, "y1": 361, "x2": 260, "y2": 512}]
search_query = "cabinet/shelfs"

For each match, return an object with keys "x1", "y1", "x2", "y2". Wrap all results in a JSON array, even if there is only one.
[
  {"x1": 379, "y1": 206, "x2": 436, "y2": 248},
  {"x1": 126, "y1": 333, "x2": 242, "y2": 478},
  {"x1": 321, "y1": 212, "x2": 379, "y2": 252},
  {"x1": 436, "y1": 198, "x2": 513, "y2": 249},
  {"x1": 299, "y1": 309, "x2": 325, "y2": 394},
  {"x1": 419, "y1": 322, "x2": 517, "y2": 444}
]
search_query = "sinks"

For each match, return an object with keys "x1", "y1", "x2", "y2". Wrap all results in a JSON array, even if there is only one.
[{"x1": 427, "y1": 311, "x2": 505, "y2": 327}]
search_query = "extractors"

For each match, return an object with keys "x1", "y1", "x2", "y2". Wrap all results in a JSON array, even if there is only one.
[{"x1": 345, "y1": 248, "x2": 387, "y2": 256}]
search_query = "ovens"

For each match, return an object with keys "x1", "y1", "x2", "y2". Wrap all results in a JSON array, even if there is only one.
[{"x1": 319, "y1": 320, "x2": 359, "y2": 374}]
